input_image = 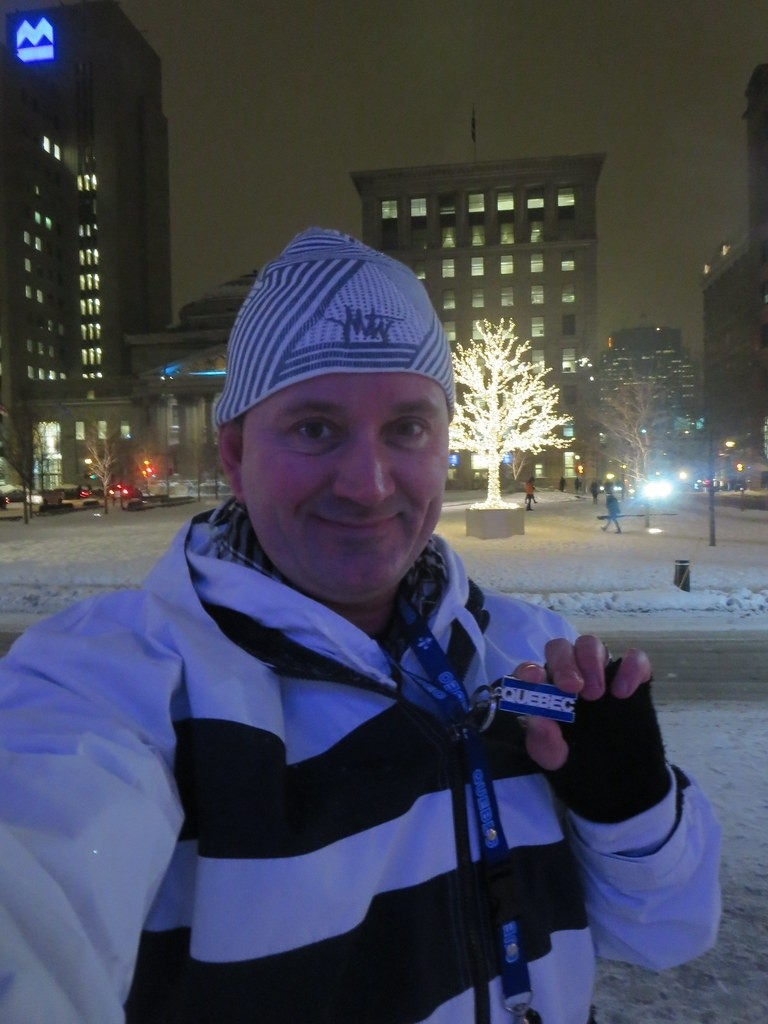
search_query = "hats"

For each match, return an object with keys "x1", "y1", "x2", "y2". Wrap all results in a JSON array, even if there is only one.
[{"x1": 215, "y1": 226, "x2": 453, "y2": 426}]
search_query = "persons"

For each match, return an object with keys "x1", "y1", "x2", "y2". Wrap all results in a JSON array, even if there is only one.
[
  {"x1": 601, "y1": 491, "x2": 623, "y2": 535},
  {"x1": 591, "y1": 478, "x2": 598, "y2": 504},
  {"x1": 558, "y1": 476, "x2": 567, "y2": 492},
  {"x1": 1, "y1": 227, "x2": 725, "y2": 1023},
  {"x1": 573, "y1": 476, "x2": 582, "y2": 493},
  {"x1": 523, "y1": 477, "x2": 537, "y2": 511}
]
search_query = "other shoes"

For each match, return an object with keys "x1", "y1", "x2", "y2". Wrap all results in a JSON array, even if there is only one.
[
  {"x1": 616, "y1": 530, "x2": 622, "y2": 534},
  {"x1": 601, "y1": 526, "x2": 605, "y2": 531}
]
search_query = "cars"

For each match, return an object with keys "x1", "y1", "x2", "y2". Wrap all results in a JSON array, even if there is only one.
[
  {"x1": 25, "y1": 491, "x2": 63, "y2": 506},
  {"x1": 108, "y1": 484, "x2": 144, "y2": 500}
]
